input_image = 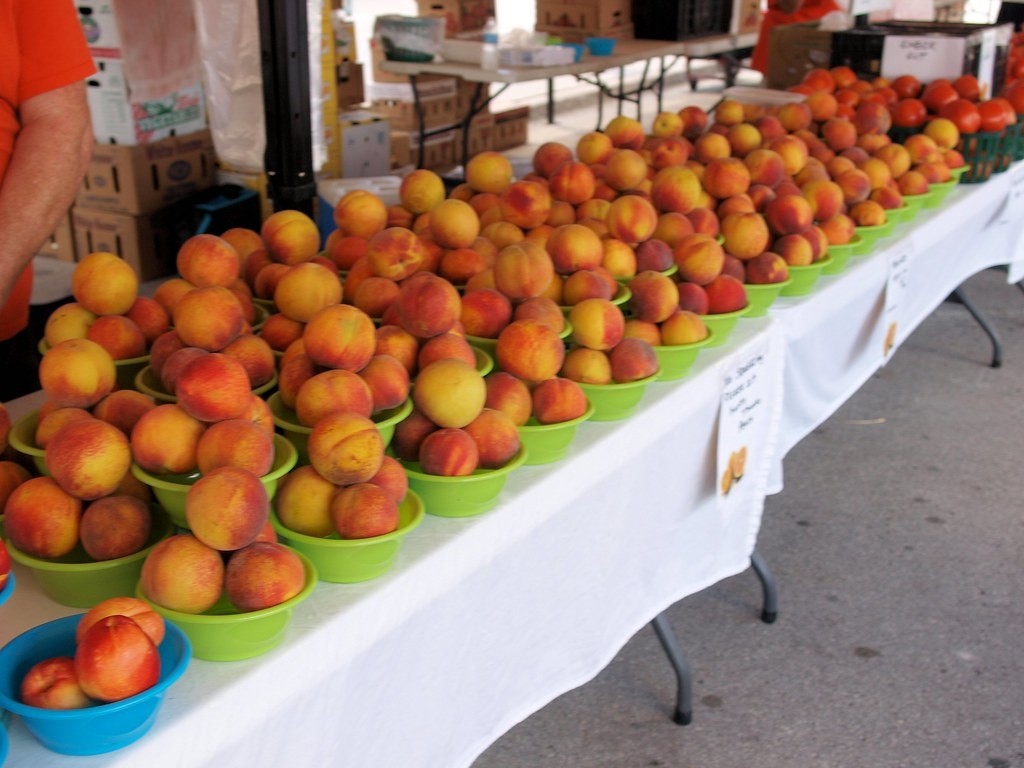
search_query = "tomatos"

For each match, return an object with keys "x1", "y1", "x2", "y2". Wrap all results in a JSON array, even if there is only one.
[{"x1": 789, "y1": 31, "x2": 1024, "y2": 179}]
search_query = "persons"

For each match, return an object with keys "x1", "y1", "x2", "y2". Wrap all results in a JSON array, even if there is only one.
[{"x1": 0, "y1": 0, "x2": 99, "y2": 404}]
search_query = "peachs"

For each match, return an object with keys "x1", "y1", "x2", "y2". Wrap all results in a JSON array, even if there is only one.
[{"x1": 0, "y1": 93, "x2": 965, "y2": 709}]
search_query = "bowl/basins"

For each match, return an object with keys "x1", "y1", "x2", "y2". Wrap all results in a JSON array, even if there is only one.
[
  {"x1": 0, "y1": 721, "x2": 9, "y2": 768},
  {"x1": 741, "y1": 276, "x2": 793, "y2": 317},
  {"x1": 0, "y1": 572, "x2": 15, "y2": 606},
  {"x1": 779, "y1": 252, "x2": 834, "y2": 296},
  {"x1": 8, "y1": 410, "x2": 50, "y2": 477},
  {"x1": 699, "y1": 301, "x2": 753, "y2": 347},
  {"x1": 131, "y1": 433, "x2": 298, "y2": 530},
  {"x1": 252, "y1": 233, "x2": 724, "y2": 339},
  {"x1": 406, "y1": 442, "x2": 529, "y2": 518},
  {"x1": 821, "y1": 200, "x2": 891, "y2": 275},
  {"x1": 877, "y1": 165, "x2": 970, "y2": 239},
  {"x1": 0, "y1": 613, "x2": 191, "y2": 756},
  {"x1": 136, "y1": 544, "x2": 317, "y2": 663},
  {"x1": 556, "y1": 38, "x2": 618, "y2": 62},
  {"x1": 516, "y1": 398, "x2": 596, "y2": 465},
  {"x1": 40, "y1": 303, "x2": 494, "y2": 455},
  {"x1": 577, "y1": 366, "x2": 663, "y2": 421},
  {"x1": 270, "y1": 489, "x2": 425, "y2": 583},
  {"x1": 4, "y1": 526, "x2": 176, "y2": 609},
  {"x1": 652, "y1": 326, "x2": 716, "y2": 381}
]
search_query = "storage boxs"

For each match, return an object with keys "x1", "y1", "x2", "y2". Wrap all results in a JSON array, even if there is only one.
[
  {"x1": 535, "y1": 0, "x2": 760, "y2": 45},
  {"x1": 153, "y1": 184, "x2": 260, "y2": 279},
  {"x1": 74, "y1": 0, "x2": 201, "y2": 57},
  {"x1": 331, "y1": 0, "x2": 528, "y2": 180},
  {"x1": 766, "y1": 13, "x2": 1013, "y2": 98},
  {"x1": 72, "y1": 206, "x2": 168, "y2": 281},
  {"x1": 79, "y1": 58, "x2": 207, "y2": 143},
  {"x1": 81, "y1": 128, "x2": 219, "y2": 214},
  {"x1": 316, "y1": 173, "x2": 402, "y2": 247}
]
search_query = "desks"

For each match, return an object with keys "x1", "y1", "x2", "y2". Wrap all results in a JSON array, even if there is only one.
[
  {"x1": 751, "y1": 157, "x2": 1024, "y2": 623},
  {"x1": 654, "y1": 23, "x2": 762, "y2": 125},
  {"x1": 0, "y1": 323, "x2": 783, "y2": 768},
  {"x1": 378, "y1": 41, "x2": 685, "y2": 180}
]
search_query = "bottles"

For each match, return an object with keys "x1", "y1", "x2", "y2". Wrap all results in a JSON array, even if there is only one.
[
  {"x1": 429, "y1": 4, "x2": 448, "y2": 65},
  {"x1": 481, "y1": 11, "x2": 498, "y2": 71}
]
